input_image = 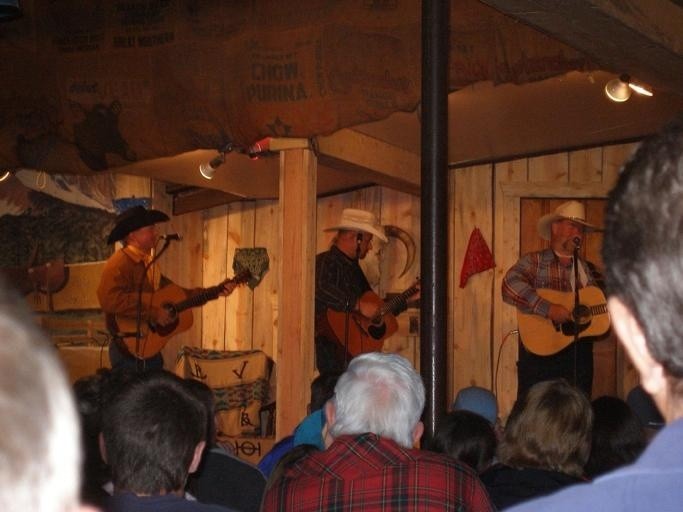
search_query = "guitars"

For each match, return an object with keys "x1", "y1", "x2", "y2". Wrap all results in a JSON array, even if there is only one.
[
  {"x1": 517, "y1": 285, "x2": 610, "y2": 355},
  {"x1": 106, "y1": 269, "x2": 253, "y2": 361},
  {"x1": 320, "y1": 281, "x2": 421, "y2": 363}
]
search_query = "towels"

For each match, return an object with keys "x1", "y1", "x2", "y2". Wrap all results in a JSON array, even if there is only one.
[{"x1": 460, "y1": 228, "x2": 496, "y2": 290}]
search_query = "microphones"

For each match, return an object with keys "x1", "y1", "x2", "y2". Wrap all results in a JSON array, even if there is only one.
[
  {"x1": 155, "y1": 232, "x2": 185, "y2": 241},
  {"x1": 117, "y1": 330, "x2": 146, "y2": 338},
  {"x1": 356, "y1": 230, "x2": 364, "y2": 246}
]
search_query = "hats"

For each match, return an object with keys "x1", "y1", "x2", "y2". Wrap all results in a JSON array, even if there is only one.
[
  {"x1": 106, "y1": 204, "x2": 171, "y2": 246},
  {"x1": 322, "y1": 207, "x2": 389, "y2": 244},
  {"x1": 449, "y1": 386, "x2": 498, "y2": 428},
  {"x1": 535, "y1": 199, "x2": 605, "y2": 241}
]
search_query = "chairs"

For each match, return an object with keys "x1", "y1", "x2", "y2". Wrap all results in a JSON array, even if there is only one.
[{"x1": 177, "y1": 344, "x2": 276, "y2": 466}]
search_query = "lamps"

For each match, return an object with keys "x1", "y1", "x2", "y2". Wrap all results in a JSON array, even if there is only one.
[
  {"x1": 199, "y1": 156, "x2": 224, "y2": 179},
  {"x1": 628, "y1": 78, "x2": 653, "y2": 96},
  {"x1": 603, "y1": 73, "x2": 631, "y2": 102}
]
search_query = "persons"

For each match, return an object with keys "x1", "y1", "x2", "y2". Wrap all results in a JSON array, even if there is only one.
[
  {"x1": 483, "y1": 380, "x2": 591, "y2": 512},
  {"x1": 501, "y1": 125, "x2": 682, "y2": 512},
  {"x1": 98, "y1": 208, "x2": 236, "y2": 377},
  {"x1": 315, "y1": 208, "x2": 390, "y2": 376},
  {"x1": 585, "y1": 398, "x2": 649, "y2": 470},
  {"x1": 250, "y1": 373, "x2": 341, "y2": 483},
  {"x1": 263, "y1": 354, "x2": 485, "y2": 512},
  {"x1": 428, "y1": 409, "x2": 497, "y2": 471},
  {"x1": 501, "y1": 200, "x2": 601, "y2": 420},
  {"x1": 95, "y1": 382, "x2": 208, "y2": 511}
]
